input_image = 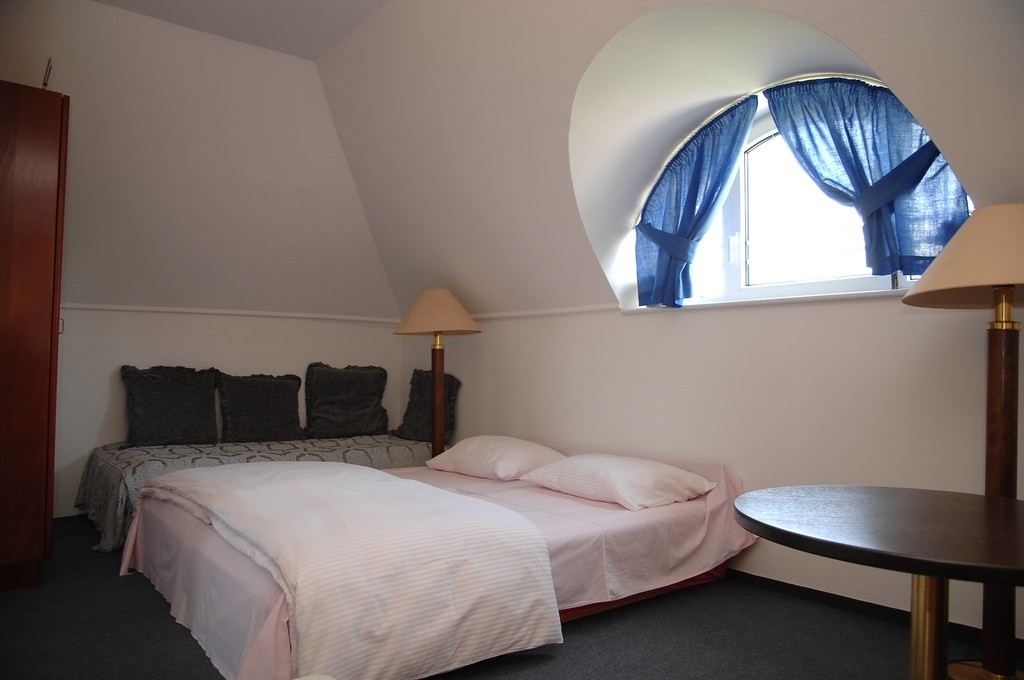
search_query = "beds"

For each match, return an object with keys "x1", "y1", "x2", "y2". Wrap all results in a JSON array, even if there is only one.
[{"x1": 119, "y1": 434, "x2": 762, "y2": 680}]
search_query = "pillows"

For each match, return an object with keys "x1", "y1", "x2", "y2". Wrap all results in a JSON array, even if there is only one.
[
  {"x1": 517, "y1": 453, "x2": 720, "y2": 512},
  {"x1": 305, "y1": 362, "x2": 389, "y2": 439},
  {"x1": 117, "y1": 364, "x2": 221, "y2": 450},
  {"x1": 390, "y1": 368, "x2": 463, "y2": 446},
  {"x1": 216, "y1": 367, "x2": 305, "y2": 444},
  {"x1": 426, "y1": 435, "x2": 568, "y2": 482}
]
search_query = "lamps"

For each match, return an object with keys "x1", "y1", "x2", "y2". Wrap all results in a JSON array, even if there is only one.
[
  {"x1": 394, "y1": 288, "x2": 483, "y2": 460},
  {"x1": 902, "y1": 203, "x2": 1024, "y2": 680}
]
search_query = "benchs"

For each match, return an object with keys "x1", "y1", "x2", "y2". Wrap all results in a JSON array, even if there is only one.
[{"x1": 73, "y1": 430, "x2": 458, "y2": 553}]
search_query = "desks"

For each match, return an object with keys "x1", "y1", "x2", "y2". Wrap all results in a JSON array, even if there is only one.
[{"x1": 734, "y1": 485, "x2": 1024, "y2": 680}]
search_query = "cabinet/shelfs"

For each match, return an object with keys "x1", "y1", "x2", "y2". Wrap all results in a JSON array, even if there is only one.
[{"x1": 0, "y1": 78, "x2": 72, "y2": 594}]
